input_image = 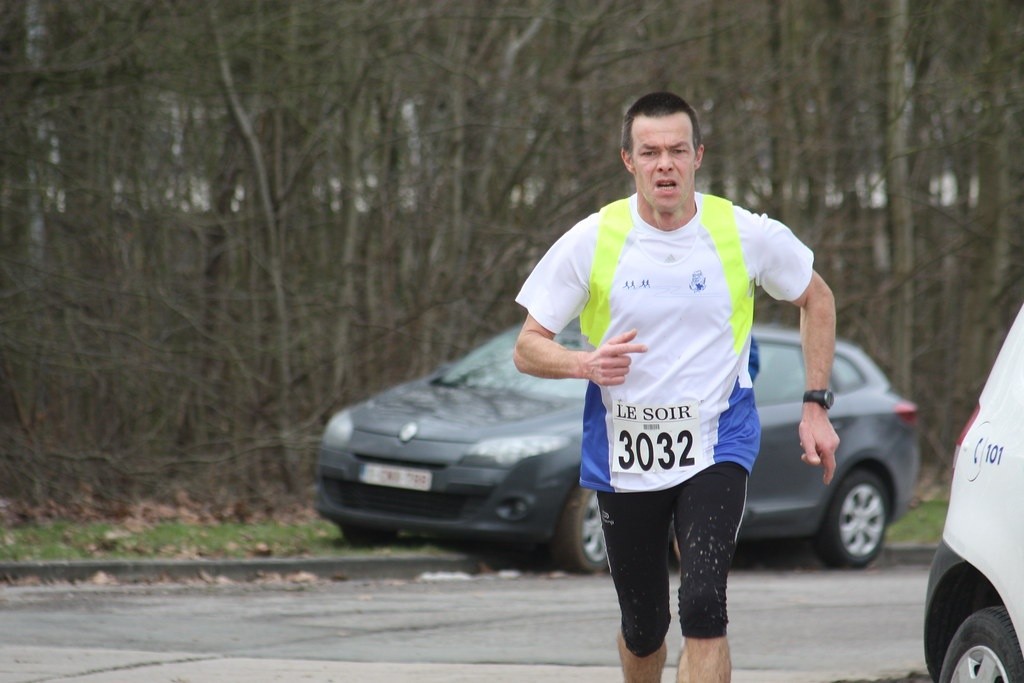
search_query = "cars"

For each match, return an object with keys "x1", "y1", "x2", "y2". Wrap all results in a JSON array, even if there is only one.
[
  {"x1": 921, "y1": 297, "x2": 1024, "y2": 682},
  {"x1": 315, "y1": 317, "x2": 922, "y2": 577}
]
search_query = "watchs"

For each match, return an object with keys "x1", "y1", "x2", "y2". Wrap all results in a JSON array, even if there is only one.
[{"x1": 803, "y1": 388, "x2": 834, "y2": 409}]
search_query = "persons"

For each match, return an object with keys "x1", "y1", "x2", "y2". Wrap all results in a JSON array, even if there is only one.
[{"x1": 512, "y1": 93, "x2": 839, "y2": 682}]
